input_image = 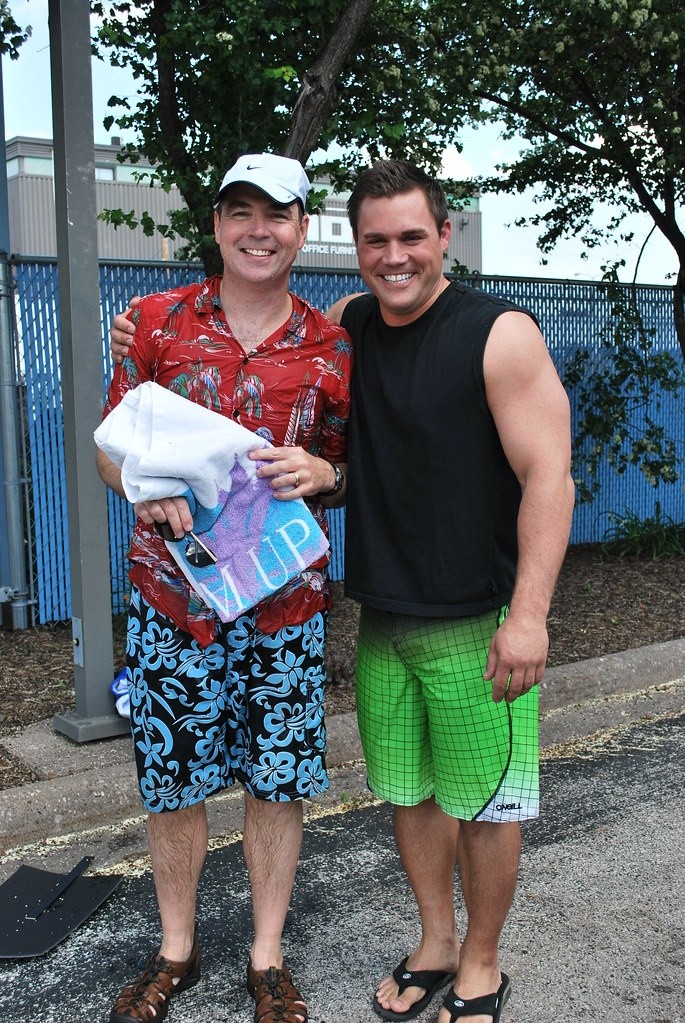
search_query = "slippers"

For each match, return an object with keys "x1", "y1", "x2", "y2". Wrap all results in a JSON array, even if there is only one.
[
  {"x1": 431, "y1": 974, "x2": 511, "y2": 1023},
  {"x1": 373, "y1": 956, "x2": 456, "y2": 1023}
]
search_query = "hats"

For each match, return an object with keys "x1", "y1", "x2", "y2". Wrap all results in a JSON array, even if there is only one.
[{"x1": 213, "y1": 153, "x2": 311, "y2": 209}]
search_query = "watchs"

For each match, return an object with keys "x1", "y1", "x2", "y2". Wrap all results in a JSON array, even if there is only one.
[{"x1": 318, "y1": 462, "x2": 344, "y2": 496}]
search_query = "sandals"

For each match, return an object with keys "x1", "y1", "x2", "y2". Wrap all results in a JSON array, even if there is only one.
[
  {"x1": 112, "y1": 943, "x2": 202, "y2": 1023},
  {"x1": 242, "y1": 950, "x2": 308, "y2": 1023}
]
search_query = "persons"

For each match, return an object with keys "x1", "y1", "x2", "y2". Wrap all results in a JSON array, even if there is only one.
[{"x1": 93, "y1": 153, "x2": 576, "y2": 1023}]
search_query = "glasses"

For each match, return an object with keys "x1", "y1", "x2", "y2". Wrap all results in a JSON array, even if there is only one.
[{"x1": 153, "y1": 517, "x2": 217, "y2": 568}]
